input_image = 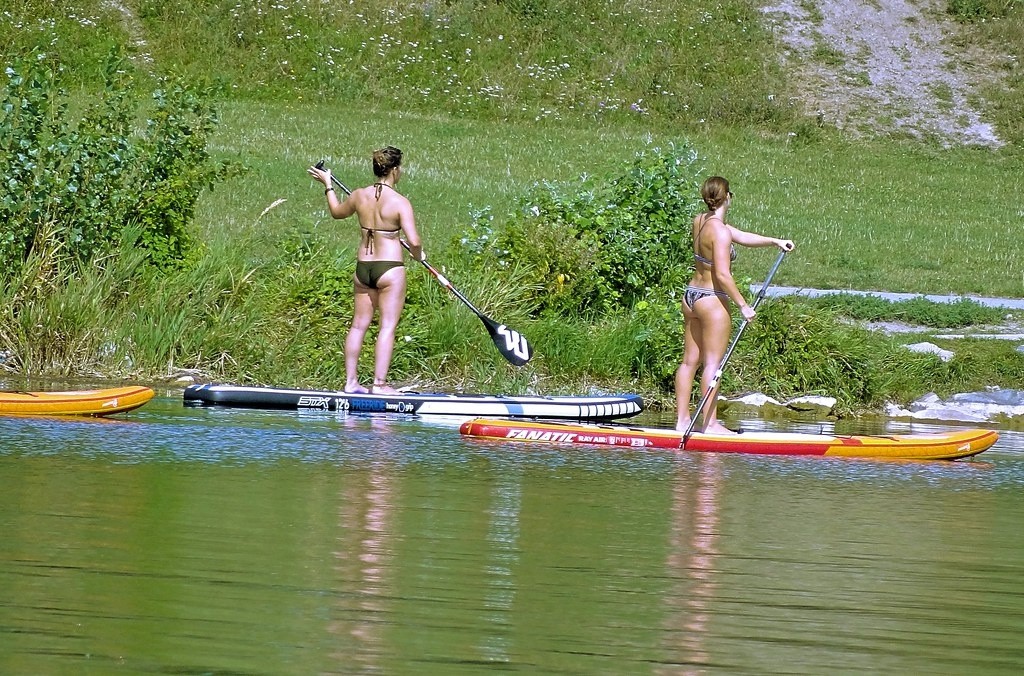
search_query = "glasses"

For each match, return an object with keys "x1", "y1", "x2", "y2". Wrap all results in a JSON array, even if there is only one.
[{"x1": 727, "y1": 190, "x2": 733, "y2": 199}]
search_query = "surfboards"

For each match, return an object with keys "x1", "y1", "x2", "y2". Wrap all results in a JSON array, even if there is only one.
[
  {"x1": 184, "y1": 382, "x2": 646, "y2": 423},
  {"x1": 461, "y1": 418, "x2": 999, "y2": 458},
  {"x1": 0, "y1": 383, "x2": 155, "y2": 419}
]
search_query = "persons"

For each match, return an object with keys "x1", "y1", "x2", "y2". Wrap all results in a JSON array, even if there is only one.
[
  {"x1": 307, "y1": 146, "x2": 426, "y2": 395},
  {"x1": 674, "y1": 176, "x2": 796, "y2": 434}
]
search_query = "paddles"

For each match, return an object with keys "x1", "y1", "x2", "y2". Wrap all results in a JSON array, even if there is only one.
[
  {"x1": 316, "y1": 160, "x2": 534, "y2": 367},
  {"x1": 680, "y1": 246, "x2": 791, "y2": 447}
]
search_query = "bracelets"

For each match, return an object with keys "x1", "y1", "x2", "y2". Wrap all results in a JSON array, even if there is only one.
[{"x1": 325, "y1": 188, "x2": 334, "y2": 195}]
